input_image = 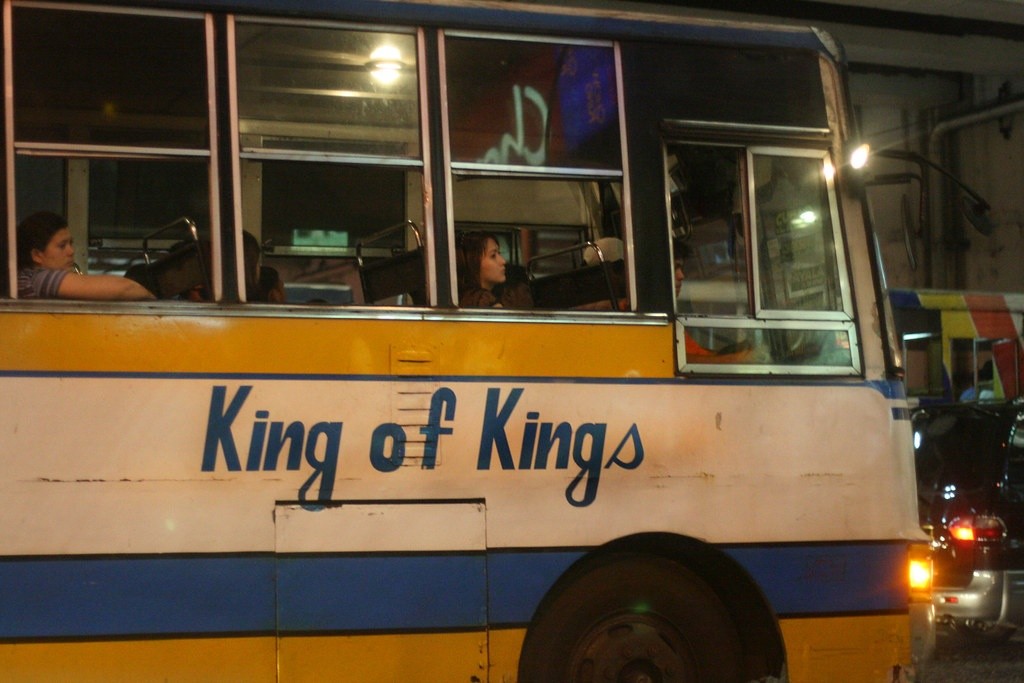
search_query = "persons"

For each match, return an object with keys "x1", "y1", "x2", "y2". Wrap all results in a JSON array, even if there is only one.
[
  {"x1": 218, "y1": 228, "x2": 284, "y2": 304},
  {"x1": 456, "y1": 230, "x2": 532, "y2": 310},
  {"x1": 672, "y1": 238, "x2": 754, "y2": 362},
  {"x1": 17, "y1": 211, "x2": 157, "y2": 300}
]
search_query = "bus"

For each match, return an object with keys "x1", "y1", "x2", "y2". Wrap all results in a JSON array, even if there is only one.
[{"x1": 0, "y1": 0, "x2": 997, "y2": 683}]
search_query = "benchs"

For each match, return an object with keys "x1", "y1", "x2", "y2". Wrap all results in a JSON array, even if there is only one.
[
  {"x1": 125, "y1": 218, "x2": 214, "y2": 302},
  {"x1": 499, "y1": 243, "x2": 619, "y2": 312},
  {"x1": 356, "y1": 220, "x2": 427, "y2": 307}
]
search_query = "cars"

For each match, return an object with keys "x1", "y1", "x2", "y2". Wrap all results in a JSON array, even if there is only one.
[{"x1": 911, "y1": 399, "x2": 1024, "y2": 590}]
